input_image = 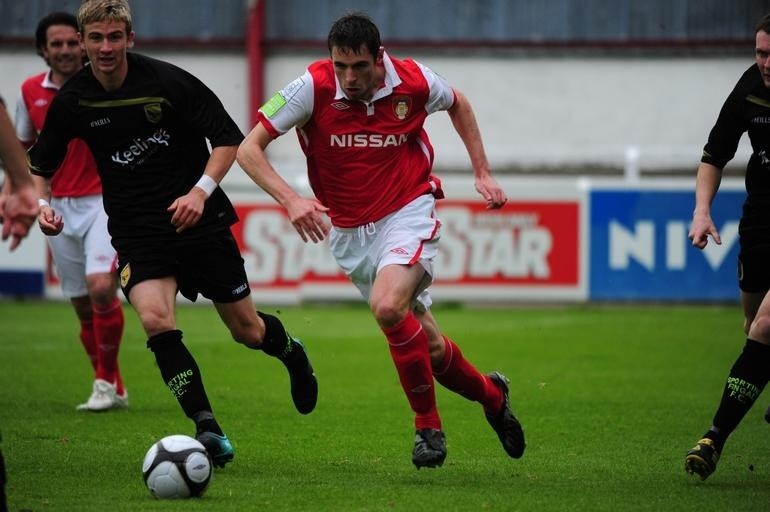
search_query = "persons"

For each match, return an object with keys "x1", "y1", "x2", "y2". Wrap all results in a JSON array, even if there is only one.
[
  {"x1": 233, "y1": 12, "x2": 527, "y2": 466},
  {"x1": 0, "y1": 0, "x2": 319, "y2": 469},
  {"x1": 683, "y1": 13, "x2": 770, "y2": 478},
  {"x1": 0, "y1": 9, "x2": 130, "y2": 412},
  {"x1": 0, "y1": 95, "x2": 41, "y2": 251}
]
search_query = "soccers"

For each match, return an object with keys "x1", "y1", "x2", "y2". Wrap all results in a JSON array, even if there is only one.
[{"x1": 143, "y1": 434, "x2": 214, "y2": 499}]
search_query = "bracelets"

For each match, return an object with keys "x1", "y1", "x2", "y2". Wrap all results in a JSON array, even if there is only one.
[{"x1": 195, "y1": 174, "x2": 217, "y2": 197}]
